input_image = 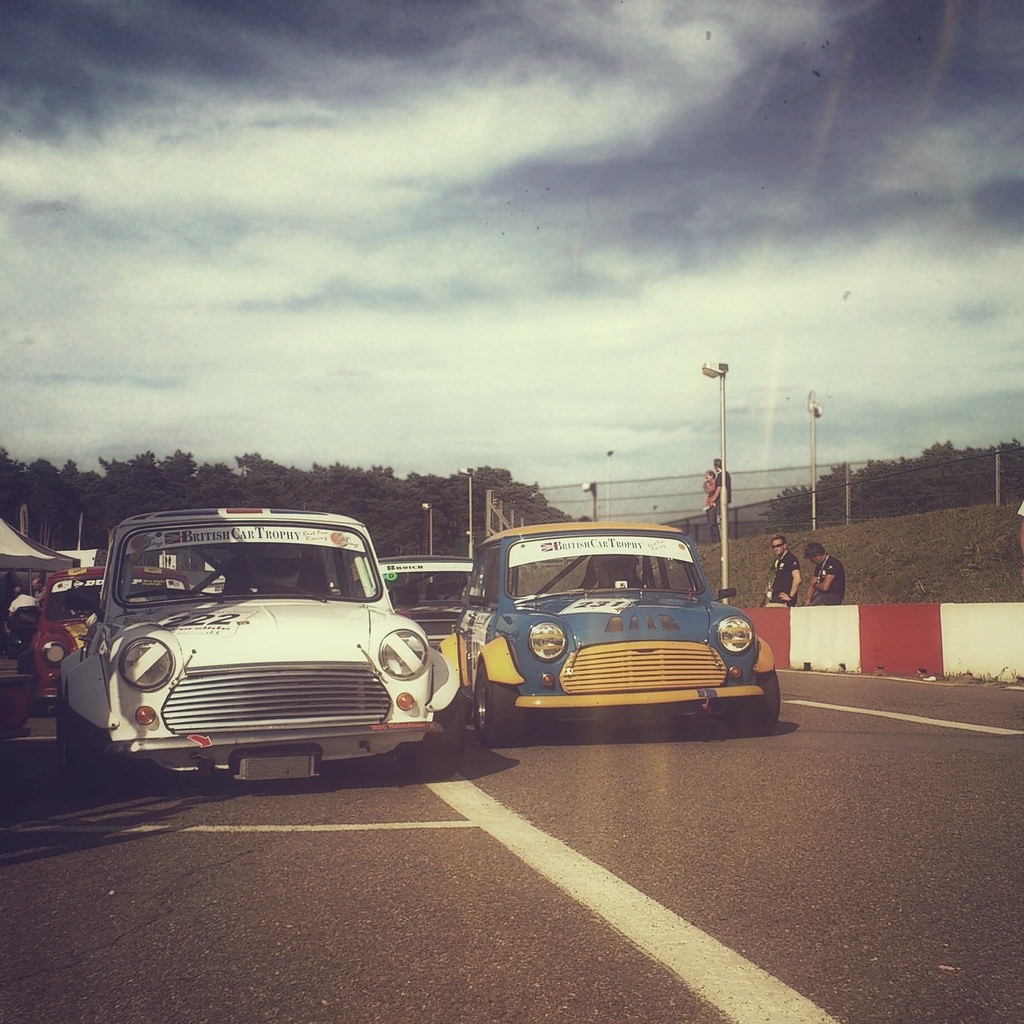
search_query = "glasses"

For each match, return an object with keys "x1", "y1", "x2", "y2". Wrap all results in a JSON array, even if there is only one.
[{"x1": 772, "y1": 544, "x2": 784, "y2": 548}]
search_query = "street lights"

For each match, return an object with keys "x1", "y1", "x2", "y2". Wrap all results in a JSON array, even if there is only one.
[
  {"x1": 806, "y1": 389, "x2": 823, "y2": 530},
  {"x1": 422, "y1": 502, "x2": 433, "y2": 555},
  {"x1": 703, "y1": 361, "x2": 730, "y2": 607},
  {"x1": 458, "y1": 467, "x2": 474, "y2": 557},
  {"x1": 606, "y1": 449, "x2": 614, "y2": 521}
]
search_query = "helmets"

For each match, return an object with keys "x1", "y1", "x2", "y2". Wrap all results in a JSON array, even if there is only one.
[
  {"x1": 594, "y1": 555, "x2": 639, "y2": 588},
  {"x1": 434, "y1": 572, "x2": 461, "y2": 601},
  {"x1": 247, "y1": 546, "x2": 302, "y2": 591}
]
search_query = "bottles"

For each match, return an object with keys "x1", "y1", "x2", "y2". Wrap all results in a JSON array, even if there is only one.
[{"x1": 767, "y1": 582, "x2": 772, "y2": 598}]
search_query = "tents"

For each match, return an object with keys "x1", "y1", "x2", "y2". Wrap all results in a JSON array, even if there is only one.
[{"x1": 0, "y1": 518, "x2": 81, "y2": 596}]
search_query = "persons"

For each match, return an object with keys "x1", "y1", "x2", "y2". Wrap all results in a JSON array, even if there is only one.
[
  {"x1": 32, "y1": 578, "x2": 48, "y2": 601},
  {"x1": 8, "y1": 586, "x2": 39, "y2": 620},
  {"x1": 711, "y1": 459, "x2": 731, "y2": 528},
  {"x1": 801, "y1": 542, "x2": 845, "y2": 606},
  {"x1": 704, "y1": 470, "x2": 720, "y2": 543},
  {"x1": 765, "y1": 535, "x2": 801, "y2": 607}
]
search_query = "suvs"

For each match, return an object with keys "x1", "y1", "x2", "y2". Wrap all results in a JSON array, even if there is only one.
[
  {"x1": 377, "y1": 554, "x2": 475, "y2": 651},
  {"x1": 27, "y1": 566, "x2": 191, "y2": 718},
  {"x1": 53, "y1": 507, "x2": 469, "y2": 794},
  {"x1": 436, "y1": 521, "x2": 781, "y2": 748}
]
